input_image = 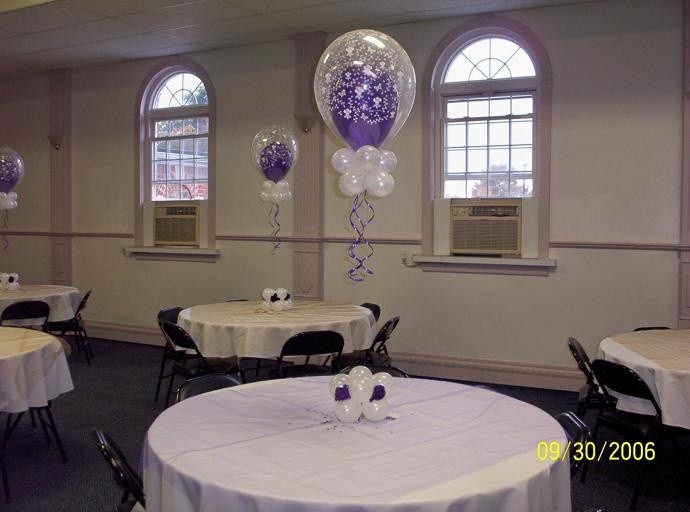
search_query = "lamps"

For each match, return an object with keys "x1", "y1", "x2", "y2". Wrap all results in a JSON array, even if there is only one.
[
  {"x1": 296, "y1": 115, "x2": 315, "y2": 134},
  {"x1": 46, "y1": 133, "x2": 63, "y2": 151}
]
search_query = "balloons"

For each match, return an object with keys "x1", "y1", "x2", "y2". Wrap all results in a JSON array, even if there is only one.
[
  {"x1": 313, "y1": 28, "x2": 417, "y2": 196},
  {"x1": 0, "y1": 146, "x2": 25, "y2": 211},
  {"x1": 329, "y1": 366, "x2": 394, "y2": 424},
  {"x1": 251, "y1": 124, "x2": 299, "y2": 203},
  {"x1": 262, "y1": 287, "x2": 294, "y2": 312},
  {"x1": 0, "y1": 272, "x2": 19, "y2": 292}
]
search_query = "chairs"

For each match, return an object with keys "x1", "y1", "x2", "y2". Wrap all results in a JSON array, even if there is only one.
[
  {"x1": 0, "y1": 300, "x2": 50, "y2": 333},
  {"x1": 361, "y1": 302, "x2": 381, "y2": 320},
  {"x1": 93, "y1": 429, "x2": 145, "y2": 511},
  {"x1": 47, "y1": 289, "x2": 93, "y2": 366},
  {"x1": 591, "y1": 358, "x2": 682, "y2": 511},
  {"x1": 557, "y1": 411, "x2": 592, "y2": 481},
  {"x1": 345, "y1": 316, "x2": 401, "y2": 365},
  {"x1": 161, "y1": 320, "x2": 208, "y2": 409},
  {"x1": 633, "y1": 327, "x2": 671, "y2": 331},
  {"x1": 269, "y1": 331, "x2": 344, "y2": 379},
  {"x1": 566, "y1": 337, "x2": 617, "y2": 441},
  {"x1": 153, "y1": 306, "x2": 198, "y2": 401}
]
